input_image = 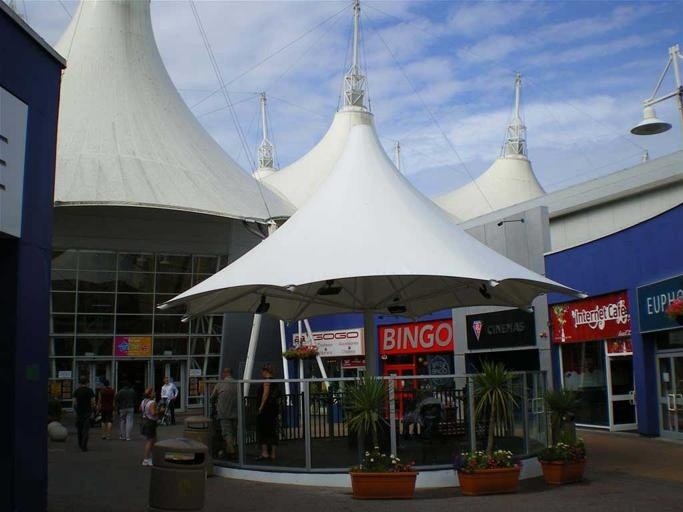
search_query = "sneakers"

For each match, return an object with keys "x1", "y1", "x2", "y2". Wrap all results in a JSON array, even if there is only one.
[
  {"x1": 142, "y1": 460, "x2": 153, "y2": 466},
  {"x1": 254, "y1": 455, "x2": 271, "y2": 461},
  {"x1": 102, "y1": 433, "x2": 131, "y2": 441}
]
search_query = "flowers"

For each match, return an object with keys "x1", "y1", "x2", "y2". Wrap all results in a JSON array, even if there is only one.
[
  {"x1": 358, "y1": 447, "x2": 416, "y2": 472},
  {"x1": 540, "y1": 440, "x2": 585, "y2": 459},
  {"x1": 457, "y1": 446, "x2": 515, "y2": 470},
  {"x1": 664, "y1": 298, "x2": 683, "y2": 320}
]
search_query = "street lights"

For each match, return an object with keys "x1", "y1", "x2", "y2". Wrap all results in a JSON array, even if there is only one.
[{"x1": 630, "y1": 43, "x2": 682, "y2": 135}]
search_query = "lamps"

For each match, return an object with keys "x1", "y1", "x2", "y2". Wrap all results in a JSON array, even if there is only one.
[
  {"x1": 479, "y1": 283, "x2": 492, "y2": 300},
  {"x1": 254, "y1": 294, "x2": 272, "y2": 313},
  {"x1": 388, "y1": 297, "x2": 407, "y2": 314},
  {"x1": 317, "y1": 280, "x2": 343, "y2": 295}
]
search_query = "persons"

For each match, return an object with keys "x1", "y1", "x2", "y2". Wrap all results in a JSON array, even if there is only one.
[
  {"x1": 96, "y1": 380, "x2": 114, "y2": 441},
  {"x1": 211, "y1": 367, "x2": 238, "y2": 455},
  {"x1": 72, "y1": 378, "x2": 93, "y2": 451},
  {"x1": 255, "y1": 362, "x2": 280, "y2": 459},
  {"x1": 116, "y1": 381, "x2": 138, "y2": 442},
  {"x1": 139, "y1": 387, "x2": 160, "y2": 466},
  {"x1": 161, "y1": 376, "x2": 179, "y2": 424}
]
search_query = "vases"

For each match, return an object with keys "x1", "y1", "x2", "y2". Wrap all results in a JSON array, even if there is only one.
[
  {"x1": 541, "y1": 459, "x2": 585, "y2": 485},
  {"x1": 456, "y1": 464, "x2": 521, "y2": 495},
  {"x1": 349, "y1": 467, "x2": 417, "y2": 500}
]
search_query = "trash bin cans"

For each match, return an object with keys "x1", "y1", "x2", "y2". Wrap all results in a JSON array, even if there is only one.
[
  {"x1": 148, "y1": 438, "x2": 208, "y2": 512},
  {"x1": 185, "y1": 416, "x2": 214, "y2": 477}
]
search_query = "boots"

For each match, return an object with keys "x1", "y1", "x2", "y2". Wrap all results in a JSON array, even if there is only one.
[{"x1": 225, "y1": 439, "x2": 235, "y2": 454}]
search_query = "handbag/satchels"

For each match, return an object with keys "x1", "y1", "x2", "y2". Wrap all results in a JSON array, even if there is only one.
[{"x1": 141, "y1": 416, "x2": 157, "y2": 437}]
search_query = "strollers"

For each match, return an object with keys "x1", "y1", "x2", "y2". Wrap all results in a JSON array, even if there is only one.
[{"x1": 156, "y1": 397, "x2": 171, "y2": 425}]
